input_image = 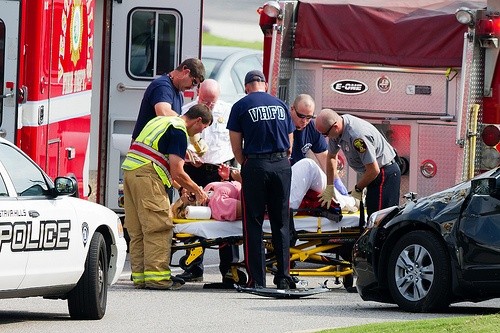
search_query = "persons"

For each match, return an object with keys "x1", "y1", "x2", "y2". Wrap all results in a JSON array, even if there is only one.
[
  {"x1": 121, "y1": 72, "x2": 400, "y2": 290},
  {"x1": 130, "y1": 58, "x2": 206, "y2": 145}
]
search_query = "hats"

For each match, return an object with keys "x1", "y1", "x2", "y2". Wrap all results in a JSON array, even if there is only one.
[{"x1": 244, "y1": 70, "x2": 266, "y2": 94}]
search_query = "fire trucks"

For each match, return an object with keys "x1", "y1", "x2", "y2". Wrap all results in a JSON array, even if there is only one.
[{"x1": 256, "y1": 0, "x2": 500, "y2": 208}]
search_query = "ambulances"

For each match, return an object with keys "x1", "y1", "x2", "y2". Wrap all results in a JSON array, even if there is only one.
[{"x1": 0, "y1": 0, "x2": 205, "y2": 218}]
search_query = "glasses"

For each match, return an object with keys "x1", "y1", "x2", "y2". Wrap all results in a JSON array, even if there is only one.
[
  {"x1": 192, "y1": 77, "x2": 198, "y2": 86},
  {"x1": 293, "y1": 105, "x2": 314, "y2": 119},
  {"x1": 321, "y1": 122, "x2": 337, "y2": 138}
]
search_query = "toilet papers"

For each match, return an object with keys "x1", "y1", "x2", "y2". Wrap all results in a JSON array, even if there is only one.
[{"x1": 185, "y1": 205, "x2": 211, "y2": 220}]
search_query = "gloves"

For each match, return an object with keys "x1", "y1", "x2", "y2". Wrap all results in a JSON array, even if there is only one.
[
  {"x1": 334, "y1": 177, "x2": 348, "y2": 195},
  {"x1": 317, "y1": 185, "x2": 338, "y2": 209},
  {"x1": 348, "y1": 187, "x2": 362, "y2": 201}
]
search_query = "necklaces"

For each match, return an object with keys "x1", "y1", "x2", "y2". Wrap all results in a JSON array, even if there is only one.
[{"x1": 168, "y1": 73, "x2": 179, "y2": 91}]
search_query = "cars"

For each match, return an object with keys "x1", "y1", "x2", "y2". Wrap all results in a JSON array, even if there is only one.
[
  {"x1": 201, "y1": 44, "x2": 263, "y2": 94},
  {"x1": 349, "y1": 164, "x2": 500, "y2": 312},
  {"x1": 0, "y1": 135, "x2": 128, "y2": 321}
]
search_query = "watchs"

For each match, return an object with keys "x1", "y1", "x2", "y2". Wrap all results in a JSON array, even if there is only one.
[{"x1": 355, "y1": 185, "x2": 362, "y2": 192}]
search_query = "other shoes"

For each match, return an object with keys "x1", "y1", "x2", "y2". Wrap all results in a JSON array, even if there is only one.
[
  {"x1": 176, "y1": 271, "x2": 203, "y2": 282},
  {"x1": 222, "y1": 270, "x2": 236, "y2": 283},
  {"x1": 166, "y1": 275, "x2": 185, "y2": 290},
  {"x1": 277, "y1": 277, "x2": 308, "y2": 289}
]
